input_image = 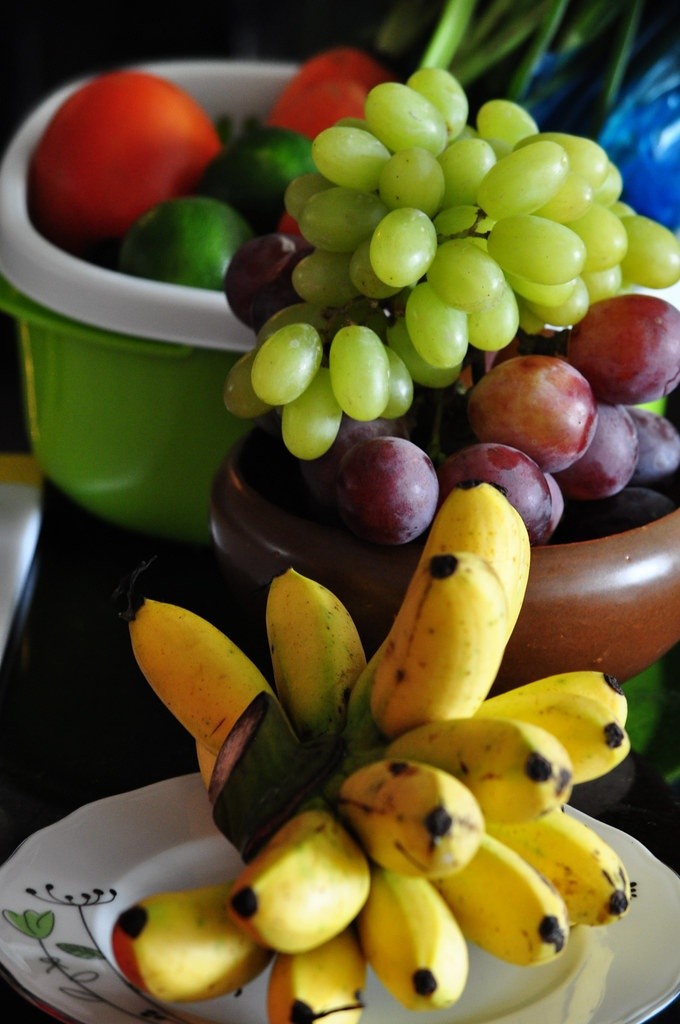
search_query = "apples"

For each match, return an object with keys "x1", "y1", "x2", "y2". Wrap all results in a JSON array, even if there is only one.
[
  {"x1": 25, "y1": 72, "x2": 222, "y2": 262},
  {"x1": 266, "y1": 47, "x2": 388, "y2": 145}
]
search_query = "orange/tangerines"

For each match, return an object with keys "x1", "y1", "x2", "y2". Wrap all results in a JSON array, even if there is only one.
[{"x1": 114, "y1": 124, "x2": 318, "y2": 291}]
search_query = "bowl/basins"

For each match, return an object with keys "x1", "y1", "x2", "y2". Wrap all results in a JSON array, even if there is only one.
[
  {"x1": 209, "y1": 373, "x2": 679, "y2": 695},
  {"x1": 0, "y1": 62, "x2": 374, "y2": 539}
]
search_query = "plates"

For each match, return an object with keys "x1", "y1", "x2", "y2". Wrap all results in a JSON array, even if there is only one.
[{"x1": 0, "y1": 760, "x2": 680, "y2": 1015}]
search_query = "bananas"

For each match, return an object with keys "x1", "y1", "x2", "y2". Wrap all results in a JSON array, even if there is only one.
[{"x1": 110, "y1": 477, "x2": 632, "y2": 1024}]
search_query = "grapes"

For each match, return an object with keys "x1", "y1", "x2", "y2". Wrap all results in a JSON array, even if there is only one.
[{"x1": 220, "y1": 66, "x2": 680, "y2": 549}]
